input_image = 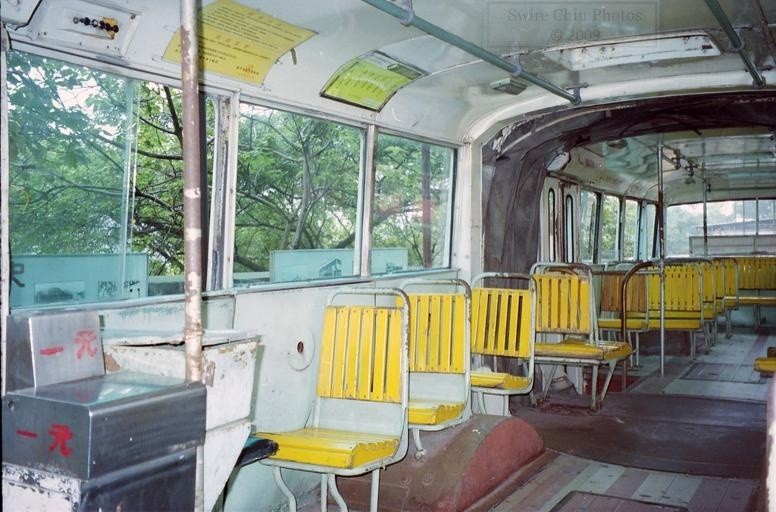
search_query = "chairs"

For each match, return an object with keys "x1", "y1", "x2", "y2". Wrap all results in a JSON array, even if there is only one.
[
  {"x1": 592, "y1": 256, "x2": 775, "y2": 378},
  {"x1": 543, "y1": 262, "x2": 631, "y2": 402},
  {"x1": 530, "y1": 260, "x2": 606, "y2": 411},
  {"x1": 251, "y1": 288, "x2": 411, "y2": 511},
  {"x1": 397, "y1": 275, "x2": 473, "y2": 459},
  {"x1": 468, "y1": 270, "x2": 537, "y2": 415}
]
